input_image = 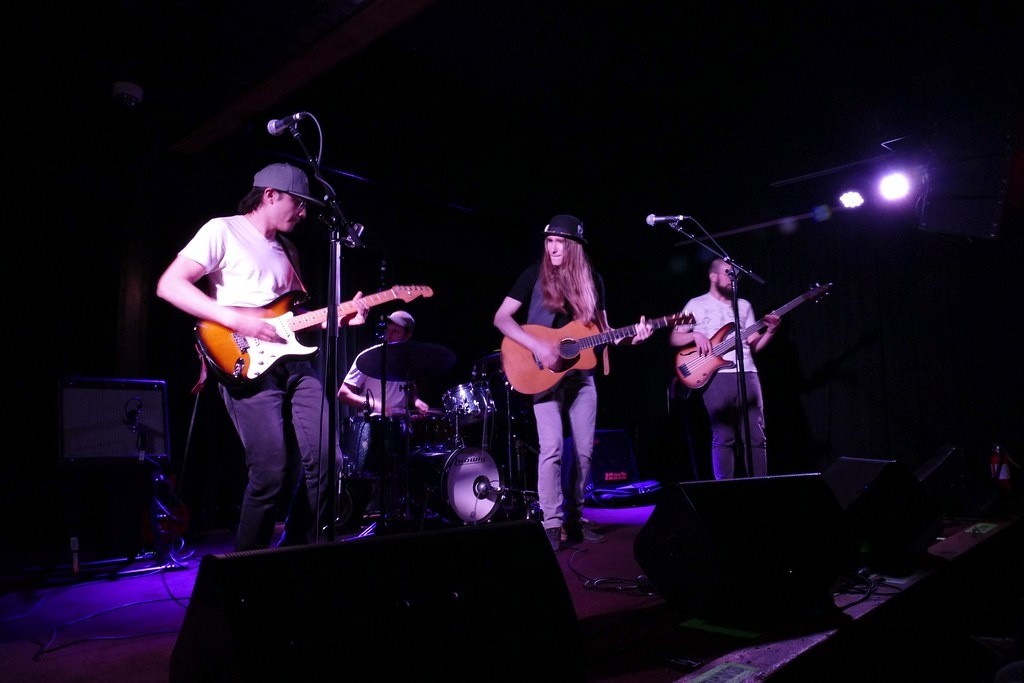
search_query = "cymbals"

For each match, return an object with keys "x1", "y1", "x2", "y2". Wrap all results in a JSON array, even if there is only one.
[
  {"x1": 357, "y1": 341, "x2": 457, "y2": 382},
  {"x1": 478, "y1": 352, "x2": 502, "y2": 362}
]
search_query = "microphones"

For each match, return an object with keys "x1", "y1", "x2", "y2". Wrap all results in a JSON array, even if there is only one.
[
  {"x1": 267, "y1": 111, "x2": 306, "y2": 136},
  {"x1": 126, "y1": 403, "x2": 142, "y2": 421},
  {"x1": 474, "y1": 481, "x2": 494, "y2": 495},
  {"x1": 646, "y1": 214, "x2": 691, "y2": 227},
  {"x1": 364, "y1": 391, "x2": 370, "y2": 408}
]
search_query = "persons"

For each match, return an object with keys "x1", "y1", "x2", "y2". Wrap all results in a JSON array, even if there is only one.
[
  {"x1": 155, "y1": 161, "x2": 370, "y2": 553},
  {"x1": 336, "y1": 310, "x2": 430, "y2": 530},
  {"x1": 492, "y1": 214, "x2": 655, "y2": 551},
  {"x1": 670, "y1": 258, "x2": 781, "y2": 481}
]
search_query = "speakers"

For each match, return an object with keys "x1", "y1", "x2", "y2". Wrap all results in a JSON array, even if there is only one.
[
  {"x1": 53, "y1": 376, "x2": 171, "y2": 466},
  {"x1": 168, "y1": 518, "x2": 579, "y2": 683},
  {"x1": 633, "y1": 447, "x2": 989, "y2": 605}
]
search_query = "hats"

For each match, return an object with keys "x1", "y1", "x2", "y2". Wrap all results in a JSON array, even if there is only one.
[
  {"x1": 253, "y1": 163, "x2": 327, "y2": 209},
  {"x1": 544, "y1": 214, "x2": 588, "y2": 246},
  {"x1": 386, "y1": 310, "x2": 415, "y2": 330}
]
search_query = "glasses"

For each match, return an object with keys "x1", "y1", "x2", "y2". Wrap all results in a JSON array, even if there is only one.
[{"x1": 289, "y1": 194, "x2": 308, "y2": 209}]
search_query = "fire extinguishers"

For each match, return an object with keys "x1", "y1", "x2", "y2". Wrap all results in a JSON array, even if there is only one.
[{"x1": 991, "y1": 442, "x2": 1012, "y2": 488}]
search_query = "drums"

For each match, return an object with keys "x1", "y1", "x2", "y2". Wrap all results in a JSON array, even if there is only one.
[
  {"x1": 401, "y1": 444, "x2": 505, "y2": 525},
  {"x1": 391, "y1": 411, "x2": 454, "y2": 457},
  {"x1": 339, "y1": 415, "x2": 413, "y2": 479},
  {"x1": 441, "y1": 380, "x2": 497, "y2": 428}
]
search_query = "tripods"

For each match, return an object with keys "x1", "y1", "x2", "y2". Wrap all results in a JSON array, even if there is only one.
[{"x1": 357, "y1": 359, "x2": 450, "y2": 537}]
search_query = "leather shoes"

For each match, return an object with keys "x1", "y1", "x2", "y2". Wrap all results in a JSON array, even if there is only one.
[
  {"x1": 566, "y1": 524, "x2": 606, "y2": 544},
  {"x1": 546, "y1": 527, "x2": 561, "y2": 552}
]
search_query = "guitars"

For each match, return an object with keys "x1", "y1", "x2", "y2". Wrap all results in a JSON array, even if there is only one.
[
  {"x1": 500, "y1": 312, "x2": 697, "y2": 395},
  {"x1": 193, "y1": 283, "x2": 434, "y2": 385},
  {"x1": 675, "y1": 282, "x2": 833, "y2": 390}
]
